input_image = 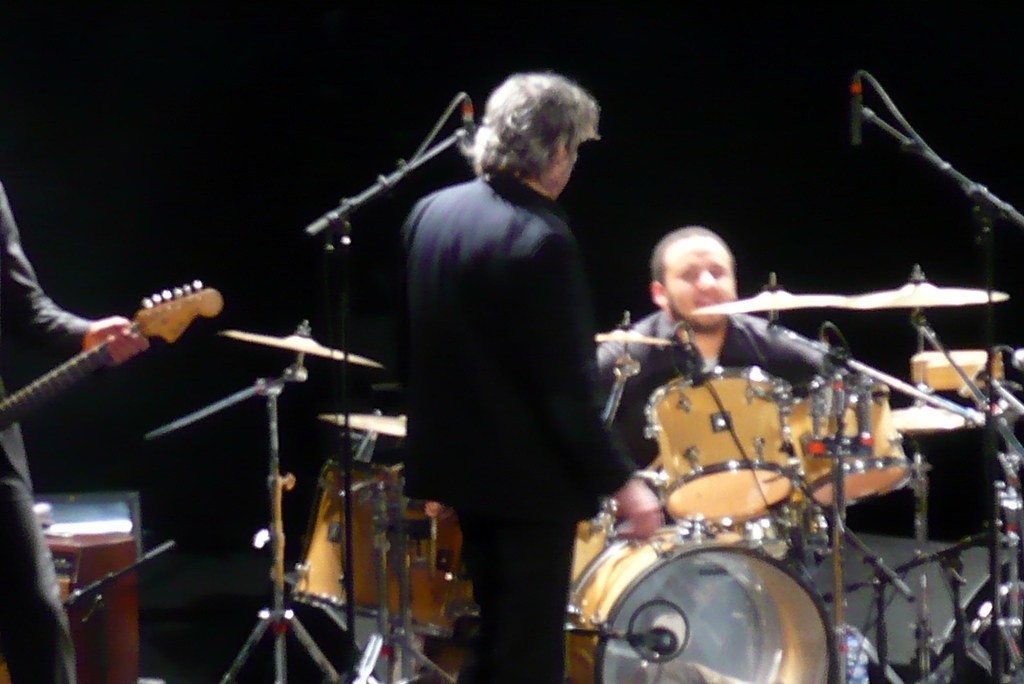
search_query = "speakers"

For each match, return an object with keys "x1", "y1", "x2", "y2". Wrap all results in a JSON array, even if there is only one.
[{"x1": 804, "y1": 530, "x2": 1017, "y2": 684}]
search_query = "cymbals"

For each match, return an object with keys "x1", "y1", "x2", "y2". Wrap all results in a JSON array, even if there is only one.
[
  {"x1": 831, "y1": 284, "x2": 1010, "y2": 311},
  {"x1": 888, "y1": 405, "x2": 979, "y2": 436},
  {"x1": 689, "y1": 292, "x2": 838, "y2": 313},
  {"x1": 595, "y1": 328, "x2": 673, "y2": 345},
  {"x1": 221, "y1": 330, "x2": 382, "y2": 371},
  {"x1": 322, "y1": 412, "x2": 407, "y2": 436}
]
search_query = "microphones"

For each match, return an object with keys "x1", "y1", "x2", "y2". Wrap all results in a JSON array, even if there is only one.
[
  {"x1": 462, "y1": 97, "x2": 477, "y2": 144},
  {"x1": 629, "y1": 627, "x2": 677, "y2": 654},
  {"x1": 683, "y1": 326, "x2": 708, "y2": 369},
  {"x1": 850, "y1": 74, "x2": 862, "y2": 146}
]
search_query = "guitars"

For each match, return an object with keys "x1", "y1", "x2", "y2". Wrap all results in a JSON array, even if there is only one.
[{"x1": 0, "y1": 279, "x2": 224, "y2": 433}]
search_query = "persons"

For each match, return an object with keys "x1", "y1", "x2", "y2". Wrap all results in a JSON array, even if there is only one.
[
  {"x1": 398, "y1": 72, "x2": 665, "y2": 684},
  {"x1": 0, "y1": 182, "x2": 150, "y2": 684},
  {"x1": 597, "y1": 227, "x2": 832, "y2": 575}
]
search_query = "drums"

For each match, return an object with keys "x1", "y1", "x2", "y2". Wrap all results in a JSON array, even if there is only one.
[
  {"x1": 781, "y1": 378, "x2": 914, "y2": 507},
  {"x1": 564, "y1": 523, "x2": 846, "y2": 684},
  {"x1": 292, "y1": 459, "x2": 474, "y2": 635},
  {"x1": 645, "y1": 365, "x2": 795, "y2": 523},
  {"x1": 909, "y1": 349, "x2": 1005, "y2": 391}
]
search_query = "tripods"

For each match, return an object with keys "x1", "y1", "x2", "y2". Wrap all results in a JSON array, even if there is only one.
[
  {"x1": 143, "y1": 346, "x2": 457, "y2": 684},
  {"x1": 917, "y1": 384, "x2": 1024, "y2": 684}
]
